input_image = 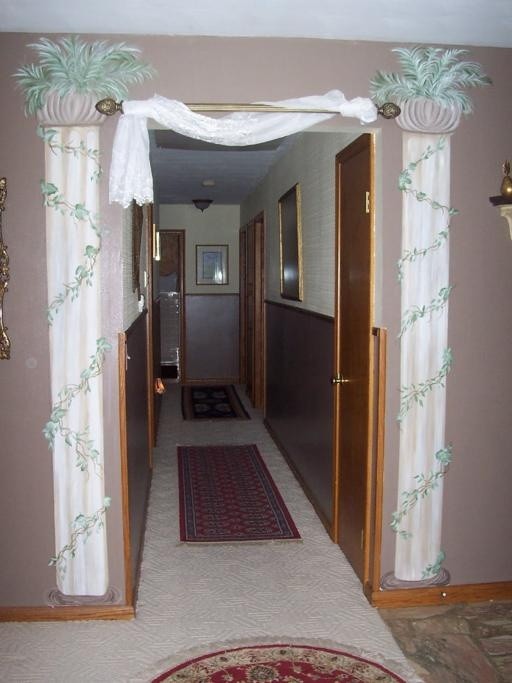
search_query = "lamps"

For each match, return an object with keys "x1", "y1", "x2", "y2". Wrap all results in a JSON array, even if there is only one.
[{"x1": 192, "y1": 199, "x2": 212, "y2": 209}]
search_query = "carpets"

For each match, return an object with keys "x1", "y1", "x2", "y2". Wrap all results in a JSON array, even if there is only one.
[
  {"x1": 180, "y1": 383, "x2": 251, "y2": 422},
  {"x1": 177, "y1": 442, "x2": 301, "y2": 542},
  {"x1": 123, "y1": 635, "x2": 424, "y2": 682}
]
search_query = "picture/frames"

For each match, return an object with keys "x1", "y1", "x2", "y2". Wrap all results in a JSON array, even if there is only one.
[
  {"x1": 152, "y1": 223, "x2": 158, "y2": 258},
  {"x1": 195, "y1": 243, "x2": 230, "y2": 286},
  {"x1": 0, "y1": 177, "x2": 13, "y2": 360},
  {"x1": 277, "y1": 181, "x2": 304, "y2": 304}
]
